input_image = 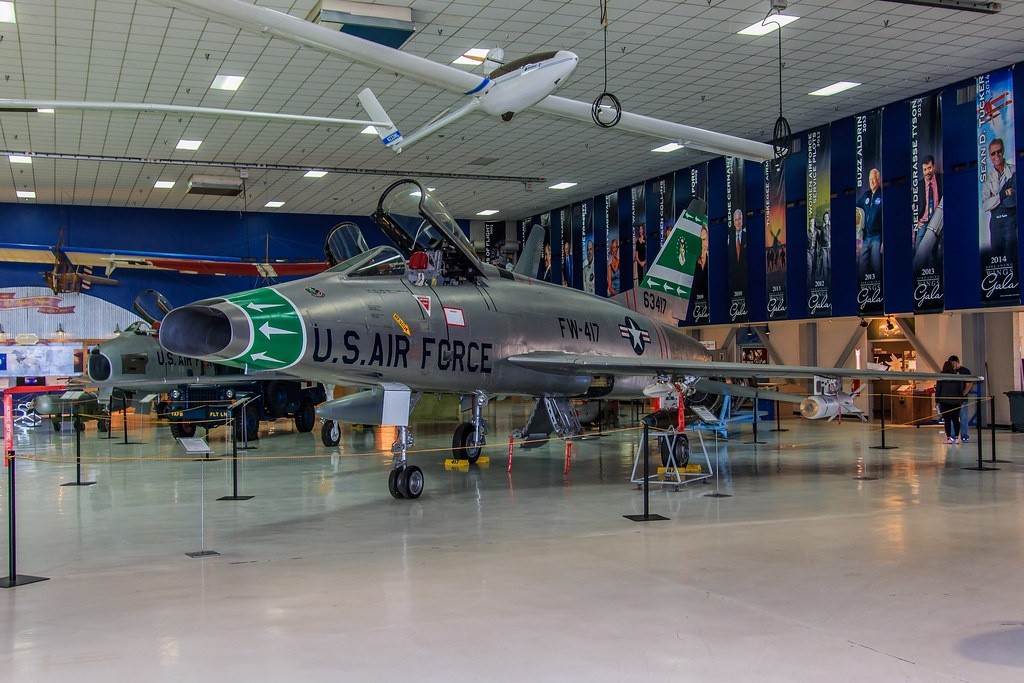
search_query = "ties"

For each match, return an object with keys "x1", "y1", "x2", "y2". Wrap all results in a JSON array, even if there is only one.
[
  {"x1": 928, "y1": 182, "x2": 933, "y2": 223},
  {"x1": 737, "y1": 234, "x2": 740, "y2": 263}
]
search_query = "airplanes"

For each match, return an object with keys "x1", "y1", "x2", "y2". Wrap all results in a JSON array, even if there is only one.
[
  {"x1": 2, "y1": 178, "x2": 985, "y2": 502},
  {"x1": 0, "y1": 1, "x2": 788, "y2": 164}
]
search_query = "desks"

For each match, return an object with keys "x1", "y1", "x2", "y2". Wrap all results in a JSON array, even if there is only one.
[{"x1": 892, "y1": 391, "x2": 932, "y2": 424}]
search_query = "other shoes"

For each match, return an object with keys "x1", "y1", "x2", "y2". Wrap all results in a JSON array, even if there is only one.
[
  {"x1": 944, "y1": 437, "x2": 959, "y2": 443},
  {"x1": 963, "y1": 438, "x2": 969, "y2": 443}
]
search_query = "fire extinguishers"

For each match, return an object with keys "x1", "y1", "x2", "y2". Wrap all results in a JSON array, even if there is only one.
[{"x1": 851, "y1": 379, "x2": 860, "y2": 396}]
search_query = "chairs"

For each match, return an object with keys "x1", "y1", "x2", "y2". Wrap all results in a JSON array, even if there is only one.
[
  {"x1": 152, "y1": 322, "x2": 160, "y2": 337},
  {"x1": 409, "y1": 252, "x2": 429, "y2": 270}
]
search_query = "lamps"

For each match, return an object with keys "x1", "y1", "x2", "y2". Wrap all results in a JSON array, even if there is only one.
[
  {"x1": 860, "y1": 317, "x2": 868, "y2": 328},
  {"x1": 114, "y1": 323, "x2": 122, "y2": 336},
  {"x1": 747, "y1": 325, "x2": 752, "y2": 336},
  {"x1": 765, "y1": 324, "x2": 771, "y2": 335},
  {"x1": 56, "y1": 323, "x2": 64, "y2": 335},
  {"x1": 886, "y1": 318, "x2": 894, "y2": 330}
]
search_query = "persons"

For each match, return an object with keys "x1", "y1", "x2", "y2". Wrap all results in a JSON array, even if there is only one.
[
  {"x1": 542, "y1": 139, "x2": 1020, "y2": 304},
  {"x1": 935, "y1": 356, "x2": 973, "y2": 444}
]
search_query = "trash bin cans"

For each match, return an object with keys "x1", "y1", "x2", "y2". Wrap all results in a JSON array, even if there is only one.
[{"x1": 1003, "y1": 390, "x2": 1024, "y2": 433}]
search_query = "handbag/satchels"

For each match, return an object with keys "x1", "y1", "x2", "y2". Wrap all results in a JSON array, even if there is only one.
[{"x1": 935, "y1": 403, "x2": 940, "y2": 414}]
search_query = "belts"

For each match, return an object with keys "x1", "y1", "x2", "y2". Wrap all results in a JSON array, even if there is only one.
[{"x1": 566, "y1": 256, "x2": 569, "y2": 276}]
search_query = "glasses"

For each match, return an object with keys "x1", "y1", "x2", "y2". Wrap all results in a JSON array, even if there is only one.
[{"x1": 990, "y1": 147, "x2": 1002, "y2": 156}]
